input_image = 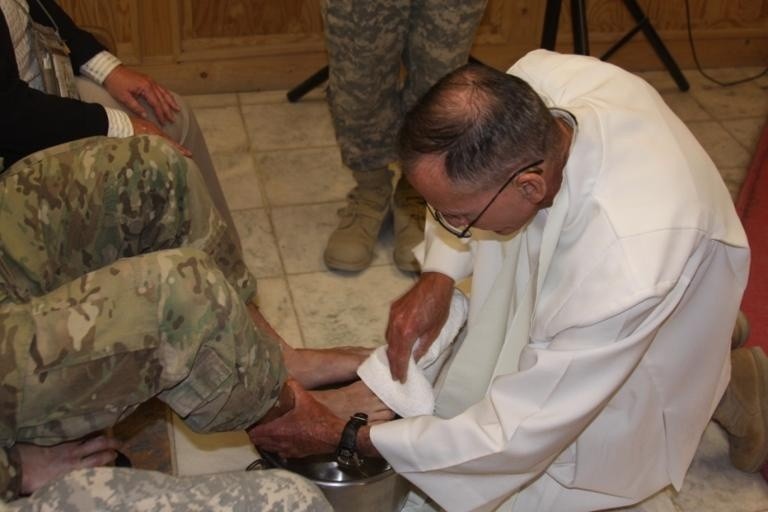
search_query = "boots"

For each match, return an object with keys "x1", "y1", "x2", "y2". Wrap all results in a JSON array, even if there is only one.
[
  {"x1": 710, "y1": 309, "x2": 768, "y2": 473},
  {"x1": 324, "y1": 166, "x2": 395, "y2": 273},
  {"x1": 392, "y1": 169, "x2": 426, "y2": 273}
]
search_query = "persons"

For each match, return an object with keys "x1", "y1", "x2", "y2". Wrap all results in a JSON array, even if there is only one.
[
  {"x1": 0, "y1": 133, "x2": 399, "y2": 506},
  {"x1": 314, "y1": 1, "x2": 492, "y2": 278},
  {"x1": 245, "y1": 47, "x2": 768, "y2": 509},
  {"x1": 0, "y1": 0, "x2": 246, "y2": 268}
]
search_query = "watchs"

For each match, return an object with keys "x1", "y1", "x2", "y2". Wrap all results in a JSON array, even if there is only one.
[{"x1": 336, "y1": 411, "x2": 376, "y2": 480}]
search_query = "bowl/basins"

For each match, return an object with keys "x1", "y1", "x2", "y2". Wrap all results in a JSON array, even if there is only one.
[{"x1": 243, "y1": 441, "x2": 415, "y2": 511}]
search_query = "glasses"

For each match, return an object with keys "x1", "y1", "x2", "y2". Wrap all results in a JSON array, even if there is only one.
[{"x1": 423, "y1": 159, "x2": 546, "y2": 238}]
